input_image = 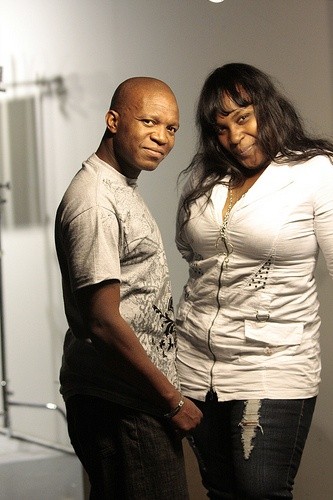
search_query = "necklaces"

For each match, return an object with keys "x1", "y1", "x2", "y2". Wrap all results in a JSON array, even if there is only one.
[{"x1": 221, "y1": 179, "x2": 249, "y2": 235}]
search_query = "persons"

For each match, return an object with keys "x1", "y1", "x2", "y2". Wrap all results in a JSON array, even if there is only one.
[
  {"x1": 54, "y1": 77, "x2": 203, "y2": 500},
  {"x1": 175, "y1": 64, "x2": 333, "y2": 500}
]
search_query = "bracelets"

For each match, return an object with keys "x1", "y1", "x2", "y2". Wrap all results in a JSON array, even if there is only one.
[{"x1": 171, "y1": 394, "x2": 183, "y2": 415}]
